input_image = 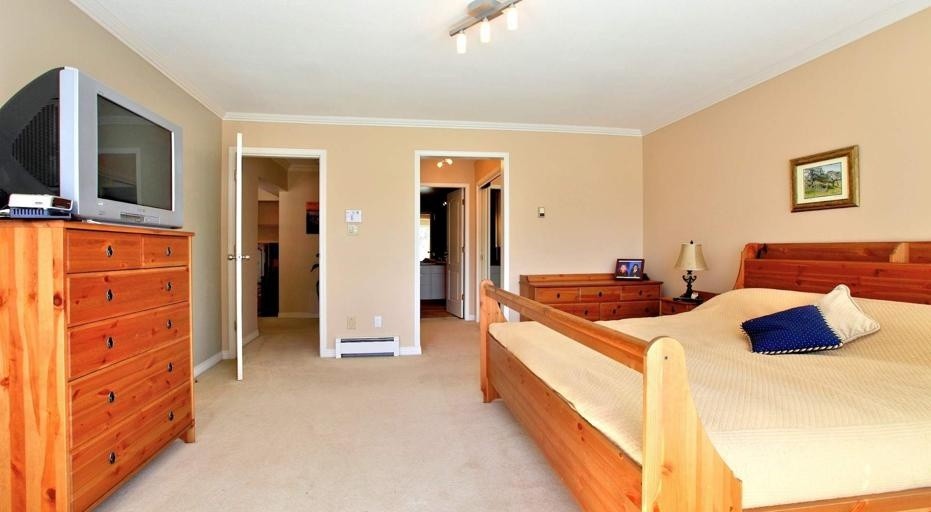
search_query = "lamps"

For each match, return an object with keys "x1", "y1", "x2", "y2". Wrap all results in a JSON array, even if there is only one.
[
  {"x1": 445, "y1": 1, "x2": 529, "y2": 56},
  {"x1": 673, "y1": 240, "x2": 711, "y2": 300},
  {"x1": 436, "y1": 158, "x2": 454, "y2": 171}
]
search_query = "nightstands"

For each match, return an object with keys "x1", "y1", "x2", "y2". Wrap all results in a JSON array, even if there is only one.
[{"x1": 661, "y1": 296, "x2": 706, "y2": 316}]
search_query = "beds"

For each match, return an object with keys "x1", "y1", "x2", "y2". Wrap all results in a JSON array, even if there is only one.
[{"x1": 477, "y1": 244, "x2": 931, "y2": 509}]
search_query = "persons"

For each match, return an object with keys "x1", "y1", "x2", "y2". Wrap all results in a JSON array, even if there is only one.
[
  {"x1": 629, "y1": 263, "x2": 641, "y2": 276},
  {"x1": 617, "y1": 263, "x2": 628, "y2": 276}
]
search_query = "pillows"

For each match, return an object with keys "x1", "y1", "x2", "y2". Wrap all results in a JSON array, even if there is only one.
[
  {"x1": 739, "y1": 303, "x2": 843, "y2": 356},
  {"x1": 815, "y1": 283, "x2": 881, "y2": 344}
]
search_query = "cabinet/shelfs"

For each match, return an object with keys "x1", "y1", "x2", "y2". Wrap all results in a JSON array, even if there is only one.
[
  {"x1": 420, "y1": 264, "x2": 445, "y2": 300},
  {"x1": 520, "y1": 272, "x2": 663, "y2": 322},
  {"x1": 0, "y1": 220, "x2": 197, "y2": 512}
]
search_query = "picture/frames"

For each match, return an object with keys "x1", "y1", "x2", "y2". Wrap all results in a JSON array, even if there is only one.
[
  {"x1": 614, "y1": 255, "x2": 644, "y2": 280},
  {"x1": 789, "y1": 143, "x2": 863, "y2": 213}
]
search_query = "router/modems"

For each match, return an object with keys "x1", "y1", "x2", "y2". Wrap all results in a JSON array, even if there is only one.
[{"x1": 9, "y1": 208, "x2": 71, "y2": 220}]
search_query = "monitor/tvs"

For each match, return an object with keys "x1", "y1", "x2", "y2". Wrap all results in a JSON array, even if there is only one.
[{"x1": 0, "y1": 66, "x2": 184, "y2": 231}]
